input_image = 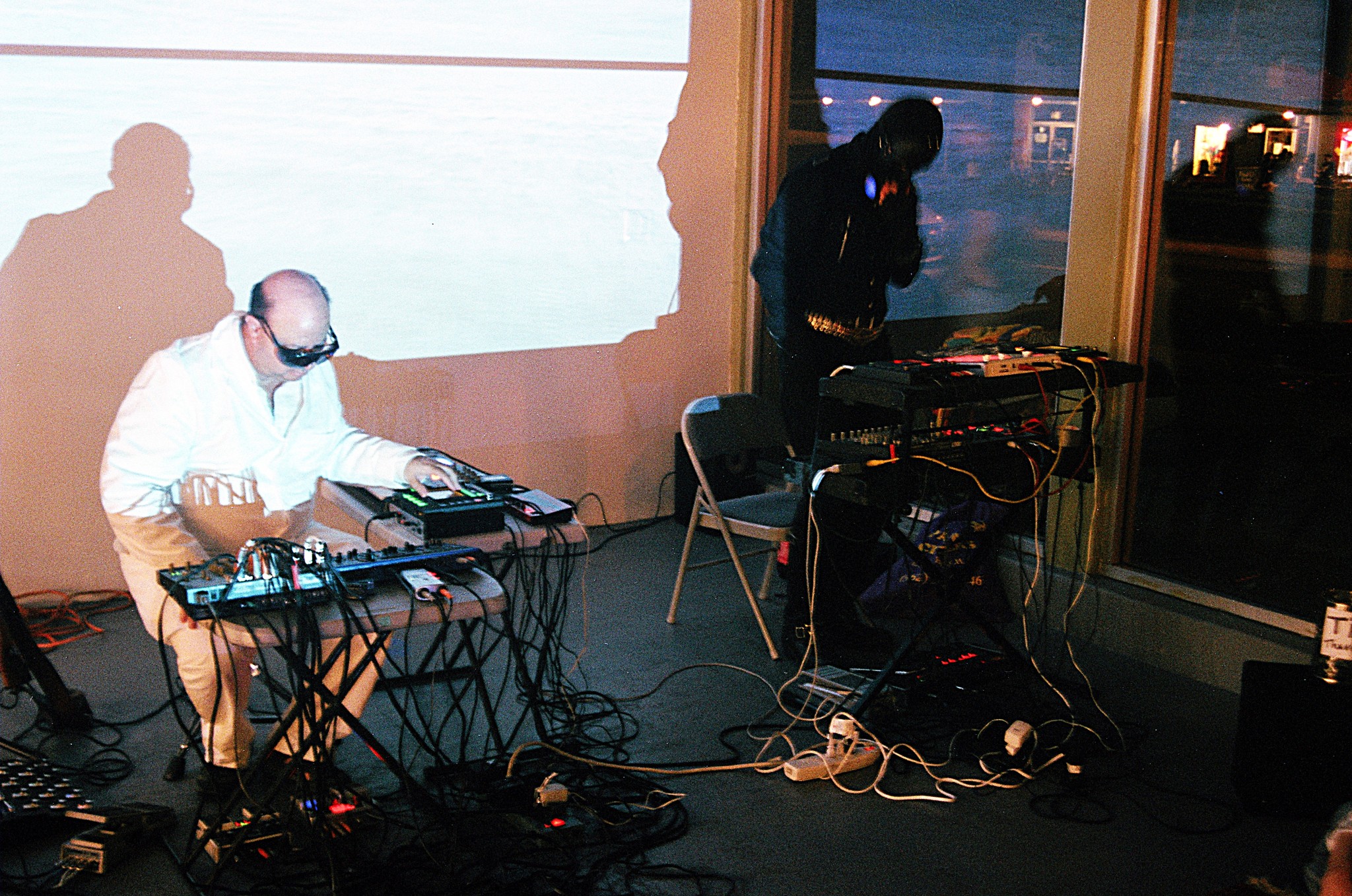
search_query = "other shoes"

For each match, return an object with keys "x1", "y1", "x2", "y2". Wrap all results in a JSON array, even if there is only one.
[
  {"x1": 781, "y1": 618, "x2": 842, "y2": 673},
  {"x1": 194, "y1": 763, "x2": 239, "y2": 796},
  {"x1": 827, "y1": 608, "x2": 896, "y2": 648},
  {"x1": 265, "y1": 750, "x2": 350, "y2": 797}
]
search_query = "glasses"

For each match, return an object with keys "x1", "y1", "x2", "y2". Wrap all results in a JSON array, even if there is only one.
[{"x1": 246, "y1": 311, "x2": 339, "y2": 367}]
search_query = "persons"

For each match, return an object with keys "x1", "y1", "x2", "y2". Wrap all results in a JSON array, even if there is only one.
[
  {"x1": 1253, "y1": 145, "x2": 1337, "y2": 185},
  {"x1": 100, "y1": 270, "x2": 464, "y2": 809},
  {"x1": 749, "y1": 97, "x2": 944, "y2": 667}
]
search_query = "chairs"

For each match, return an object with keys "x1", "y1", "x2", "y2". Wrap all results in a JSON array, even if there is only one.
[{"x1": 663, "y1": 391, "x2": 809, "y2": 662}]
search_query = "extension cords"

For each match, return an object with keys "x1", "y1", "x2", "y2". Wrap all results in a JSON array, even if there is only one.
[{"x1": 783, "y1": 744, "x2": 877, "y2": 780}]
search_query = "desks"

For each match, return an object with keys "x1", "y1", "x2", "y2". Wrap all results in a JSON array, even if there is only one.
[
  {"x1": 158, "y1": 567, "x2": 545, "y2": 896},
  {"x1": 789, "y1": 440, "x2": 1100, "y2": 728},
  {"x1": 322, "y1": 469, "x2": 587, "y2": 782}
]
